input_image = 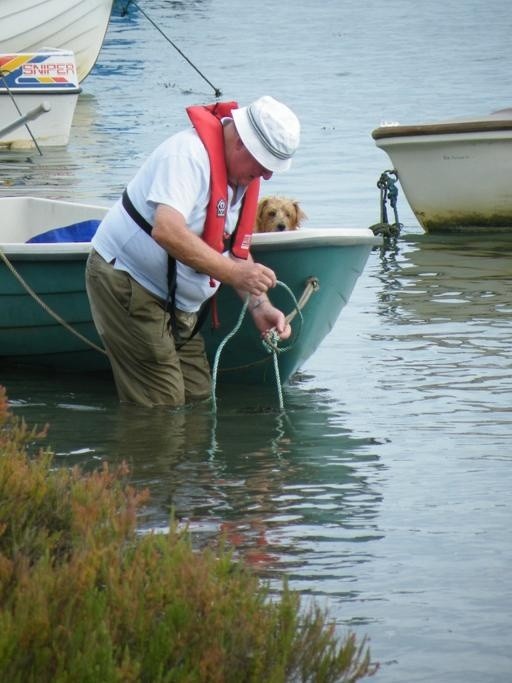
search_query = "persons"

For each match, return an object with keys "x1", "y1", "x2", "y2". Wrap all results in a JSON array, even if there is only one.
[{"x1": 85, "y1": 94, "x2": 303, "y2": 486}]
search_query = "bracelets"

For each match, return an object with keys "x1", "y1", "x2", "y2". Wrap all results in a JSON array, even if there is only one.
[{"x1": 246, "y1": 297, "x2": 269, "y2": 309}]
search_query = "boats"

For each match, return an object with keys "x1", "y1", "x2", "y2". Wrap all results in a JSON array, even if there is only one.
[
  {"x1": 371, "y1": 108, "x2": 511, "y2": 238},
  {"x1": 0, "y1": 48, "x2": 83, "y2": 153},
  {"x1": 0, "y1": 194, "x2": 375, "y2": 388},
  {"x1": 6, "y1": 377, "x2": 378, "y2": 628},
  {"x1": 0, "y1": 1, "x2": 115, "y2": 84},
  {"x1": 387, "y1": 246, "x2": 512, "y2": 383}
]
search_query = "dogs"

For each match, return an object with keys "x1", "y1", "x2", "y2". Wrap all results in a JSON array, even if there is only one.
[{"x1": 255, "y1": 196, "x2": 308, "y2": 233}]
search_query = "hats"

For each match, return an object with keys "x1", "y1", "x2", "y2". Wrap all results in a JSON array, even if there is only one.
[{"x1": 231, "y1": 96, "x2": 300, "y2": 171}]
